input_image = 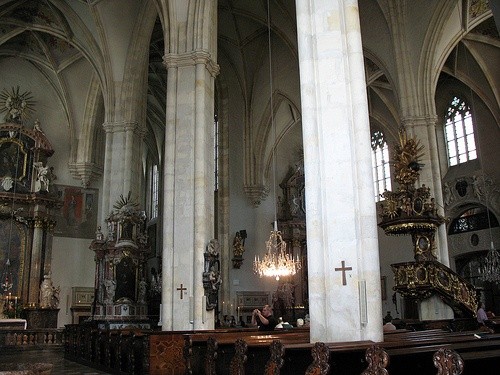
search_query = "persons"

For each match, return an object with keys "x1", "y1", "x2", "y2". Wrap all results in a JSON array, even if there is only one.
[
  {"x1": 275, "y1": 317, "x2": 284, "y2": 328},
  {"x1": 477, "y1": 302, "x2": 488, "y2": 326},
  {"x1": 234, "y1": 232, "x2": 245, "y2": 256},
  {"x1": 279, "y1": 316, "x2": 294, "y2": 328},
  {"x1": 252, "y1": 304, "x2": 279, "y2": 331},
  {"x1": 384, "y1": 316, "x2": 396, "y2": 330}
]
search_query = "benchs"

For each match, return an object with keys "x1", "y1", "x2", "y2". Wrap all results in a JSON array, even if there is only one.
[{"x1": 61, "y1": 319, "x2": 500, "y2": 375}]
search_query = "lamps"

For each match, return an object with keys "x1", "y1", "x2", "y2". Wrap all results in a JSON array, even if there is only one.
[{"x1": 252, "y1": 220, "x2": 302, "y2": 281}]
